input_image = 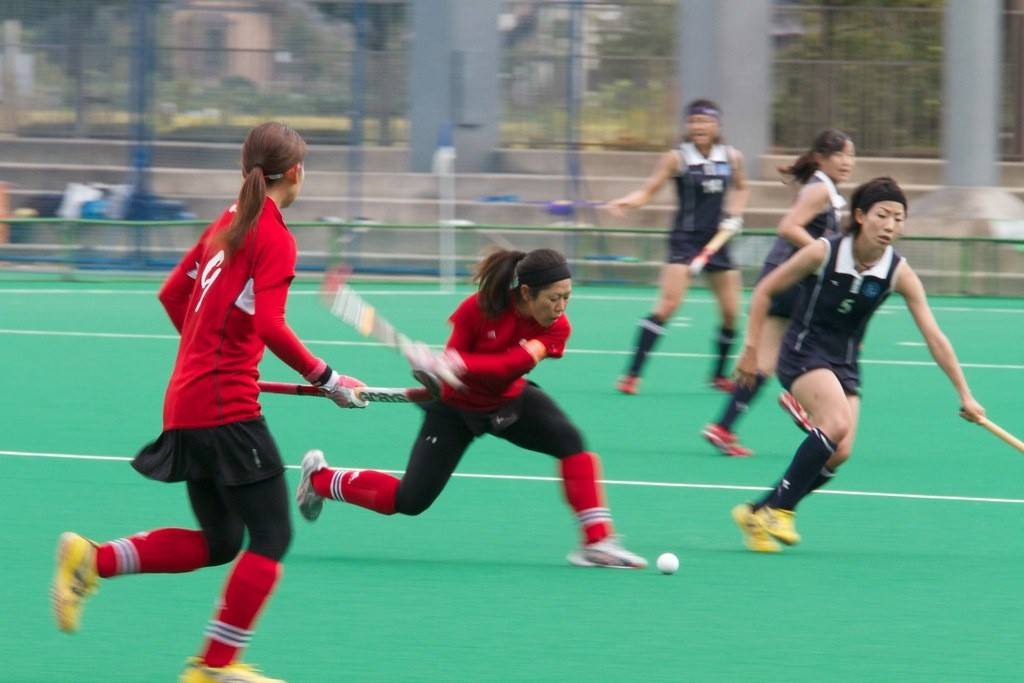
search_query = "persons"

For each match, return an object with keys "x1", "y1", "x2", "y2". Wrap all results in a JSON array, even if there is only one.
[
  {"x1": 295, "y1": 245, "x2": 645, "y2": 569},
  {"x1": 728, "y1": 173, "x2": 987, "y2": 554},
  {"x1": 700, "y1": 127, "x2": 856, "y2": 458},
  {"x1": 603, "y1": 98, "x2": 747, "y2": 400},
  {"x1": 47, "y1": 121, "x2": 374, "y2": 683}
]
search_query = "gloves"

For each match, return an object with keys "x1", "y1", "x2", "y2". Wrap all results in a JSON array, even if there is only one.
[{"x1": 304, "y1": 357, "x2": 370, "y2": 409}]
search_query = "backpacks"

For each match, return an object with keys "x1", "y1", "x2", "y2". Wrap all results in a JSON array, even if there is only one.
[{"x1": 23, "y1": 183, "x2": 197, "y2": 221}]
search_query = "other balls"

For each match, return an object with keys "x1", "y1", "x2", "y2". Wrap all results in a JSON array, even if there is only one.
[{"x1": 654, "y1": 552, "x2": 680, "y2": 575}]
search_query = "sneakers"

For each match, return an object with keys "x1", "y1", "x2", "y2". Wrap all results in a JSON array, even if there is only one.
[
  {"x1": 297, "y1": 450, "x2": 328, "y2": 522},
  {"x1": 54, "y1": 533, "x2": 99, "y2": 634},
  {"x1": 700, "y1": 423, "x2": 752, "y2": 457},
  {"x1": 179, "y1": 656, "x2": 286, "y2": 683},
  {"x1": 713, "y1": 377, "x2": 738, "y2": 393},
  {"x1": 565, "y1": 534, "x2": 648, "y2": 569},
  {"x1": 778, "y1": 391, "x2": 814, "y2": 434},
  {"x1": 757, "y1": 506, "x2": 801, "y2": 545},
  {"x1": 731, "y1": 504, "x2": 781, "y2": 553},
  {"x1": 616, "y1": 375, "x2": 639, "y2": 394}
]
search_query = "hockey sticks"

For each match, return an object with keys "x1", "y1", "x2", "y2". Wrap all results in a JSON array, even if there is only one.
[
  {"x1": 255, "y1": 367, "x2": 444, "y2": 404},
  {"x1": 958, "y1": 405, "x2": 1024, "y2": 453},
  {"x1": 317, "y1": 262, "x2": 470, "y2": 395}
]
